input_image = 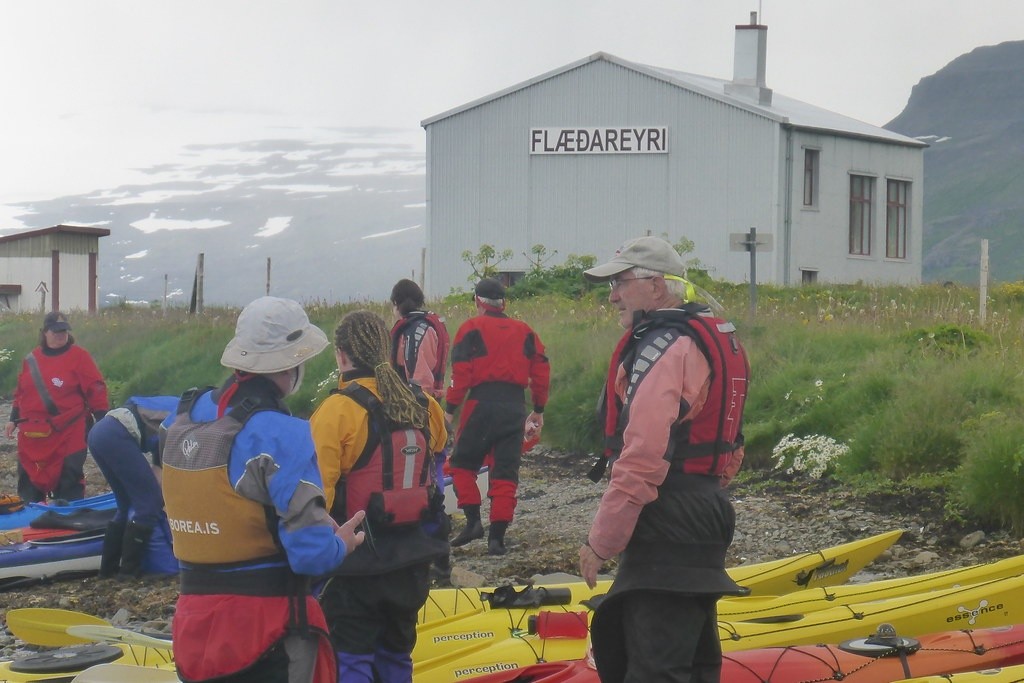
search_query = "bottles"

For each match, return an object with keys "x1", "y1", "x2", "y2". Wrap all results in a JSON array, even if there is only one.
[
  {"x1": 539, "y1": 588, "x2": 571, "y2": 605},
  {"x1": 528, "y1": 610, "x2": 588, "y2": 637}
]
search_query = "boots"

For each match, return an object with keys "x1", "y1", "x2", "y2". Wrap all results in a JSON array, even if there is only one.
[
  {"x1": 450, "y1": 504, "x2": 483, "y2": 547},
  {"x1": 487, "y1": 521, "x2": 508, "y2": 555},
  {"x1": 115, "y1": 524, "x2": 152, "y2": 584},
  {"x1": 99, "y1": 521, "x2": 121, "y2": 578}
]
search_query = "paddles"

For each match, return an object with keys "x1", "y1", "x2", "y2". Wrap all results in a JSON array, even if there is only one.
[{"x1": 5, "y1": 607, "x2": 172, "y2": 651}]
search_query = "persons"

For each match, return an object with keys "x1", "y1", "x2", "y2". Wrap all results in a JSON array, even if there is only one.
[
  {"x1": 309, "y1": 310, "x2": 447, "y2": 683},
  {"x1": 6, "y1": 311, "x2": 110, "y2": 503},
  {"x1": 390, "y1": 279, "x2": 450, "y2": 495},
  {"x1": 580, "y1": 236, "x2": 751, "y2": 683},
  {"x1": 446, "y1": 279, "x2": 550, "y2": 554},
  {"x1": 159, "y1": 297, "x2": 366, "y2": 683},
  {"x1": 88, "y1": 396, "x2": 182, "y2": 581}
]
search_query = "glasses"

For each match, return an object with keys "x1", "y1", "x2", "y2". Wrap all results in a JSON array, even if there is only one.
[{"x1": 609, "y1": 276, "x2": 653, "y2": 289}]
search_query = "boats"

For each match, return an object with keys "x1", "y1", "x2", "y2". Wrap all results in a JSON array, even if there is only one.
[
  {"x1": 0, "y1": 431, "x2": 555, "y2": 591},
  {"x1": 0, "y1": 529, "x2": 1024, "y2": 682}
]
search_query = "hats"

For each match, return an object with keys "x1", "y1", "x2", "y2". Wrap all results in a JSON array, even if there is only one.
[
  {"x1": 220, "y1": 297, "x2": 329, "y2": 373},
  {"x1": 583, "y1": 237, "x2": 685, "y2": 281},
  {"x1": 472, "y1": 279, "x2": 504, "y2": 302},
  {"x1": 44, "y1": 312, "x2": 71, "y2": 331}
]
